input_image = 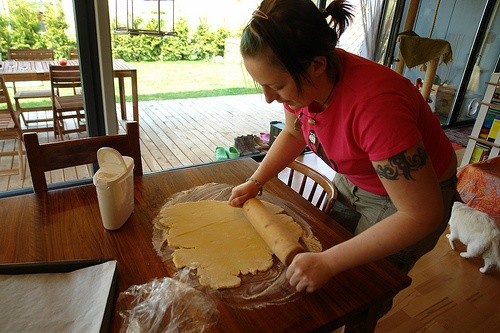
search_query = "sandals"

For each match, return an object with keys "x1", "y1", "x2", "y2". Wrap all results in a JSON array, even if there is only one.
[
  {"x1": 215, "y1": 145, "x2": 229, "y2": 160},
  {"x1": 226, "y1": 145, "x2": 240, "y2": 159}
]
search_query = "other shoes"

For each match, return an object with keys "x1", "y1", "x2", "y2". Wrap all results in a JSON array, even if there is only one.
[
  {"x1": 252, "y1": 134, "x2": 262, "y2": 151},
  {"x1": 256, "y1": 136, "x2": 270, "y2": 149}
]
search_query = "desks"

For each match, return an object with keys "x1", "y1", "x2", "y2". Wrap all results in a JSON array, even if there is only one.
[
  {"x1": 455, "y1": 156, "x2": 500, "y2": 221},
  {"x1": 0, "y1": 59, "x2": 138, "y2": 123},
  {"x1": 0, "y1": 156, "x2": 413, "y2": 333}
]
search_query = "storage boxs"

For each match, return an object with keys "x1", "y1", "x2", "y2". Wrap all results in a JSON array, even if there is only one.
[{"x1": 469, "y1": 145, "x2": 489, "y2": 165}]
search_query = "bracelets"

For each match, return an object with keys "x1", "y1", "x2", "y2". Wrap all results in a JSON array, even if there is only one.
[{"x1": 246, "y1": 177, "x2": 263, "y2": 197}]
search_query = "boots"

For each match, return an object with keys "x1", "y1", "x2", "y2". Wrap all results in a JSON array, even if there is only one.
[
  {"x1": 244, "y1": 133, "x2": 259, "y2": 154},
  {"x1": 233, "y1": 134, "x2": 253, "y2": 155}
]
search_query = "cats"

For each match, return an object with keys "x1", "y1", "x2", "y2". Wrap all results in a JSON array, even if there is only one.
[{"x1": 445, "y1": 202, "x2": 500, "y2": 273}]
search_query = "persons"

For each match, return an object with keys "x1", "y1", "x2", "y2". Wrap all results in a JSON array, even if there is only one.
[{"x1": 228, "y1": 0, "x2": 458, "y2": 319}]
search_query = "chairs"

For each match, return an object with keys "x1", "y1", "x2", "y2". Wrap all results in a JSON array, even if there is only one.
[
  {"x1": 68, "y1": 49, "x2": 85, "y2": 126},
  {"x1": 277, "y1": 161, "x2": 338, "y2": 214},
  {"x1": 0, "y1": 74, "x2": 29, "y2": 179},
  {"x1": 49, "y1": 64, "x2": 86, "y2": 140},
  {"x1": 8, "y1": 49, "x2": 64, "y2": 133},
  {"x1": 22, "y1": 120, "x2": 143, "y2": 193}
]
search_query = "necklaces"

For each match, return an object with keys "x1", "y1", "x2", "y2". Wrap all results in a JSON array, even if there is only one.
[{"x1": 293, "y1": 78, "x2": 336, "y2": 131}]
